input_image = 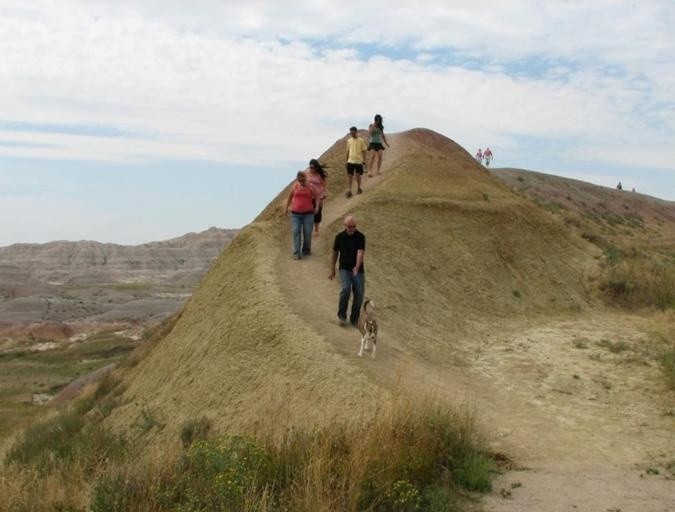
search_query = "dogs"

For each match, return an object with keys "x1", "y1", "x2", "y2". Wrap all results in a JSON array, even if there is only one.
[{"x1": 358, "y1": 299, "x2": 379, "y2": 359}]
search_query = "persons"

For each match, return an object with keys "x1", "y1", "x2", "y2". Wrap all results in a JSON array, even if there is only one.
[
  {"x1": 617, "y1": 181, "x2": 623, "y2": 190},
  {"x1": 346, "y1": 125, "x2": 367, "y2": 197},
  {"x1": 483, "y1": 147, "x2": 494, "y2": 167},
  {"x1": 367, "y1": 115, "x2": 389, "y2": 177},
  {"x1": 285, "y1": 171, "x2": 318, "y2": 261},
  {"x1": 476, "y1": 149, "x2": 483, "y2": 164},
  {"x1": 305, "y1": 158, "x2": 326, "y2": 232},
  {"x1": 328, "y1": 215, "x2": 366, "y2": 326}
]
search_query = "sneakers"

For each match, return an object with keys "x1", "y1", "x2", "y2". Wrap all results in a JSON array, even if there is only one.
[
  {"x1": 294, "y1": 254, "x2": 302, "y2": 260},
  {"x1": 347, "y1": 189, "x2": 362, "y2": 196},
  {"x1": 338, "y1": 318, "x2": 359, "y2": 327}
]
